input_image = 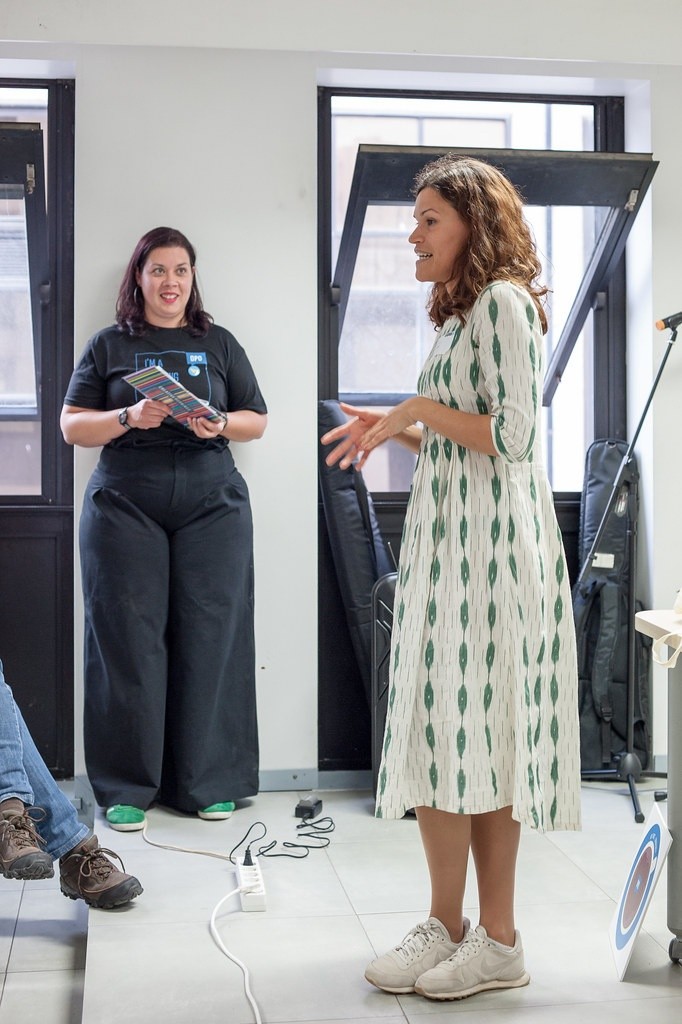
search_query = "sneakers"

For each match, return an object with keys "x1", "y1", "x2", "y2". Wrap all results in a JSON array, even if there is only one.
[
  {"x1": 365, "y1": 916, "x2": 471, "y2": 992},
  {"x1": 0, "y1": 806, "x2": 54, "y2": 880},
  {"x1": 197, "y1": 799, "x2": 236, "y2": 819},
  {"x1": 415, "y1": 925, "x2": 531, "y2": 1001},
  {"x1": 106, "y1": 801, "x2": 146, "y2": 831},
  {"x1": 58, "y1": 835, "x2": 143, "y2": 908}
]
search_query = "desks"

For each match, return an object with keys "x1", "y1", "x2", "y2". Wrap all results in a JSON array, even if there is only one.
[{"x1": 633, "y1": 608, "x2": 680, "y2": 962}]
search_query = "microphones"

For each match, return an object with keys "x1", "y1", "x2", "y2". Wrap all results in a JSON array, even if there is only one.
[{"x1": 656, "y1": 312, "x2": 682, "y2": 330}]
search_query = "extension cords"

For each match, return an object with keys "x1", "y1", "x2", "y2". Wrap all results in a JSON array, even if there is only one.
[{"x1": 235, "y1": 856, "x2": 267, "y2": 912}]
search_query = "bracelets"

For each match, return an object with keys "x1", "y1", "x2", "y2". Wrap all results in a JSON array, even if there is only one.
[{"x1": 223, "y1": 419, "x2": 228, "y2": 429}]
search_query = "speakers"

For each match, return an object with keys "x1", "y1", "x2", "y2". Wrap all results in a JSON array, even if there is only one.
[{"x1": 370, "y1": 572, "x2": 417, "y2": 817}]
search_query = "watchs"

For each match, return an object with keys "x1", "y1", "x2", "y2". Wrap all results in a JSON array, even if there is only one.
[{"x1": 118, "y1": 407, "x2": 132, "y2": 430}]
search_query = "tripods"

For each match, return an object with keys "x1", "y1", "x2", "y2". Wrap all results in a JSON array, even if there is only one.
[{"x1": 573, "y1": 326, "x2": 679, "y2": 823}]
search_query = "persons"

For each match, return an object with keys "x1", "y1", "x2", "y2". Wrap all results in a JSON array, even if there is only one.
[
  {"x1": 58, "y1": 226, "x2": 268, "y2": 833},
  {"x1": 319, "y1": 157, "x2": 581, "y2": 1000},
  {"x1": 0, "y1": 659, "x2": 142, "y2": 910}
]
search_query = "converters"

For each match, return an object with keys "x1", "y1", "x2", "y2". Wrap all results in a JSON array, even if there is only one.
[{"x1": 295, "y1": 798, "x2": 322, "y2": 819}]
